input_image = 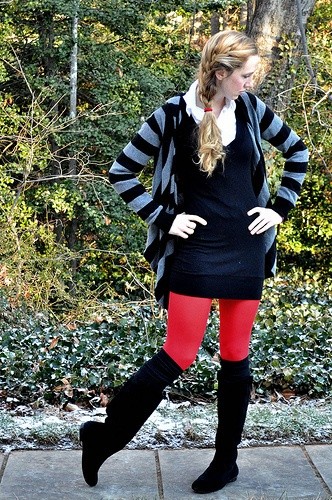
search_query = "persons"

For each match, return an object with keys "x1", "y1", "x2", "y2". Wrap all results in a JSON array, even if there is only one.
[{"x1": 80, "y1": 29, "x2": 309, "y2": 495}]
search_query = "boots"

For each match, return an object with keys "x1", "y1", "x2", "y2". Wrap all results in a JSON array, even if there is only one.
[
  {"x1": 82, "y1": 371, "x2": 170, "y2": 487},
  {"x1": 191, "y1": 371, "x2": 253, "y2": 495}
]
